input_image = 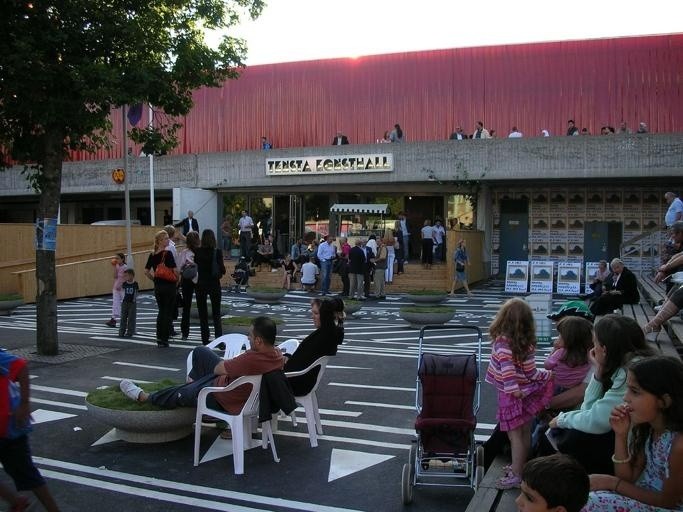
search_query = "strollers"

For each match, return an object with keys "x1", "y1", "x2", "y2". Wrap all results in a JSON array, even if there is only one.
[{"x1": 397, "y1": 325, "x2": 484, "y2": 508}]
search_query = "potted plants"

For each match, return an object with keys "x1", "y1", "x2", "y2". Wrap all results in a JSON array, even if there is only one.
[
  {"x1": 337, "y1": 298, "x2": 365, "y2": 321},
  {"x1": 0, "y1": 293, "x2": 25, "y2": 317},
  {"x1": 403, "y1": 288, "x2": 449, "y2": 308},
  {"x1": 244, "y1": 285, "x2": 290, "y2": 305},
  {"x1": 215, "y1": 314, "x2": 287, "y2": 347},
  {"x1": 176, "y1": 299, "x2": 232, "y2": 327},
  {"x1": 397, "y1": 304, "x2": 458, "y2": 332},
  {"x1": 82, "y1": 378, "x2": 197, "y2": 445}
]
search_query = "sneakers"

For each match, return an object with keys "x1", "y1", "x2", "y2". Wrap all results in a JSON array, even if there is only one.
[
  {"x1": 201, "y1": 339, "x2": 210, "y2": 345},
  {"x1": 105, "y1": 318, "x2": 116, "y2": 327},
  {"x1": 125, "y1": 330, "x2": 135, "y2": 337},
  {"x1": 501, "y1": 462, "x2": 512, "y2": 473},
  {"x1": 6, "y1": 495, "x2": 30, "y2": 511},
  {"x1": 119, "y1": 379, "x2": 143, "y2": 401},
  {"x1": 223, "y1": 255, "x2": 474, "y2": 301},
  {"x1": 576, "y1": 293, "x2": 586, "y2": 301},
  {"x1": 156, "y1": 331, "x2": 179, "y2": 347},
  {"x1": 182, "y1": 333, "x2": 188, "y2": 340},
  {"x1": 217, "y1": 343, "x2": 225, "y2": 350},
  {"x1": 494, "y1": 472, "x2": 521, "y2": 489},
  {"x1": 119, "y1": 329, "x2": 125, "y2": 338}
]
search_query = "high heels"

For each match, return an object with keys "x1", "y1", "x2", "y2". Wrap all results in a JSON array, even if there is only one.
[{"x1": 640, "y1": 305, "x2": 676, "y2": 342}]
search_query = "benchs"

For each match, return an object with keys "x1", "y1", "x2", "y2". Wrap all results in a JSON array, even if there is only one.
[
  {"x1": 466, "y1": 456, "x2": 521, "y2": 511},
  {"x1": 614, "y1": 274, "x2": 682, "y2": 362}
]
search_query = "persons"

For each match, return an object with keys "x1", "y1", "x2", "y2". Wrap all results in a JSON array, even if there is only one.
[
  {"x1": 262, "y1": 136, "x2": 271, "y2": 149},
  {"x1": 451, "y1": 120, "x2": 649, "y2": 140},
  {"x1": 128, "y1": 146, "x2": 162, "y2": 158},
  {"x1": 390, "y1": 124, "x2": 404, "y2": 143},
  {"x1": 332, "y1": 131, "x2": 349, "y2": 144},
  {"x1": 381, "y1": 131, "x2": 391, "y2": 143},
  {"x1": 0, "y1": 348, "x2": 60, "y2": 511}
]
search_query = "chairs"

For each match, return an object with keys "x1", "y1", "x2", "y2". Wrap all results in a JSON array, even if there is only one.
[{"x1": 182, "y1": 329, "x2": 329, "y2": 478}]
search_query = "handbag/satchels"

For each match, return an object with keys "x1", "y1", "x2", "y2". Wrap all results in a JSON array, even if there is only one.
[
  {"x1": 207, "y1": 248, "x2": 222, "y2": 279},
  {"x1": 180, "y1": 261, "x2": 197, "y2": 287},
  {"x1": 155, "y1": 250, "x2": 176, "y2": 282}
]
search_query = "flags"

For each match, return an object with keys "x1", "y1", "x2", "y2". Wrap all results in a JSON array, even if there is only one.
[{"x1": 126, "y1": 101, "x2": 141, "y2": 126}]
